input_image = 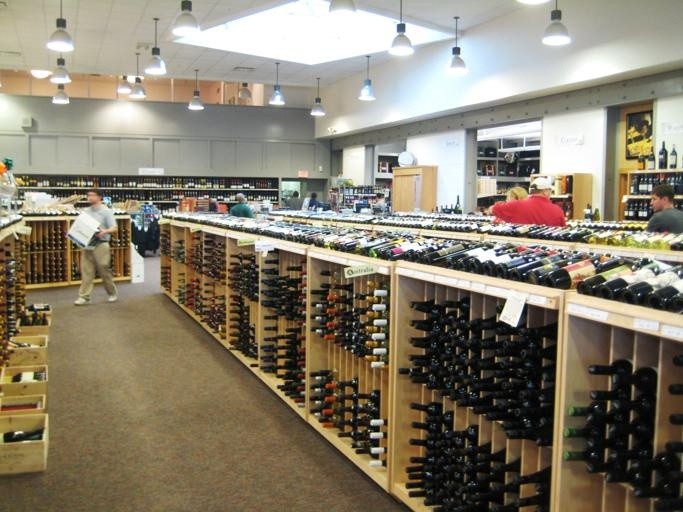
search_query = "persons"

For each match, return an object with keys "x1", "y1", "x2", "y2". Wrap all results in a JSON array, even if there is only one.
[
  {"x1": 506, "y1": 186, "x2": 528, "y2": 202},
  {"x1": 483, "y1": 177, "x2": 566, "y2": 226},
  {"x1": 74, "y1": 189, "x2": 119, "y2": 306},
  {"x1": 373, "y1": 193, "x2": 384, "y2": 213},
  {"x1": 288, "y1": 191, "x2": 302, "y2": 210},
  {"x1": 308, "y1": 192, "x2": 318, "y2": 211},
  {"x1": 646, "y1": 184, "x2": 683, "y2": 234},
  {"x1": 633, "y1": 119, "x2": 652, "y2": 142},
  {"x1": 230, "y1": 193, "x2": 256, "y2": 219}
]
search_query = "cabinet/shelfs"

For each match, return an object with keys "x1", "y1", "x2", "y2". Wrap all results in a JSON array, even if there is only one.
[
  {"x1": 1, "y1": 214, "x2": 48, "y2": 477},
  {"x1": 8, "y1": 173, "x2": 283, "y2": 288},
  {"x1": 157, "y1": 165, "x2": 683, "y2": 511}
]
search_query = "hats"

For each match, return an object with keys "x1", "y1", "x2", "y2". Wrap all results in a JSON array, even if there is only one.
[{"x1": 529, "y1": 178, "x2": 555, "y2": 189}]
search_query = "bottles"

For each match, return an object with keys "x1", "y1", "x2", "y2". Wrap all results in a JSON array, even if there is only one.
[
  {"x1": 638, "y1": 147, "x2": 646, "y2": 171},
  {"x1": 659, "y1": 142, "x2": 668, "y2": 169},
  {"x1": 670, "y1": 144, "x2": 677, "y2": 169},
  {"x1": 17, "y1": 175, "x2": 158, "y2": 202},
  {"x1": 0, "y1": 220, "x2": 132, "y2": 384},
  {"x1": 648, "y1": 147, "x2": 656, "y2": 170},
  {"x1": 161, "y1": 172, "x2": 683, "y2": 511}
]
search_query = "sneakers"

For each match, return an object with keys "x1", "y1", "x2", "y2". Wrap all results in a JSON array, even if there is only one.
[
  {"x1": 74, "y1": 298, "x2": 92, "y2": 305},
  {"x1": 109, "y1": 289, "x2": 118, "y2": 302}
]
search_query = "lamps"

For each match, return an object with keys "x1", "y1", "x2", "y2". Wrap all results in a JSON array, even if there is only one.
[
  {"x1": 50, "y1": 84, "x2": 70, "y2": 105},
  {"x1": 542, "y1": 1, "x2": 571, "y2": 46},
  {"x1": 119, "y1": 75, "x2": 131, "y2": 93},
  {"x1": 172, "y1": 2, "x2": 200, "y2": 35},
  {"x1": 143, "y1": 19, "x2": 168, "y2": 76},
  {"x1": 48, "y1": 51, "x2": 72, "y2": 86},
  {"x1": 390, "y1": 1, "x2": 413, "y2": 57},
  {"x1": 269, "y1": 63, "x2": 286, "y2": 105},
  {"x1": 310, "y1": 77, "x2": 325, "y2": 116},
  {"x1": 187, "y1": 70, "x2": 206, "y2": 111},
  {"x1": 48, "y1": 2, "x2": 74, "y2": 52},
  {"x1": 128, "y1": 53, "x2": 146, "y2": 99},
  {"x1": 237, "y1": 82, "x2": 252, "y2": 99},
  {"x1": 449, "y1": 16, "x2": 465, "y2": 70},
  {"x1": 358, "y1": 56, "x2": 376, "y2": 101}
]
